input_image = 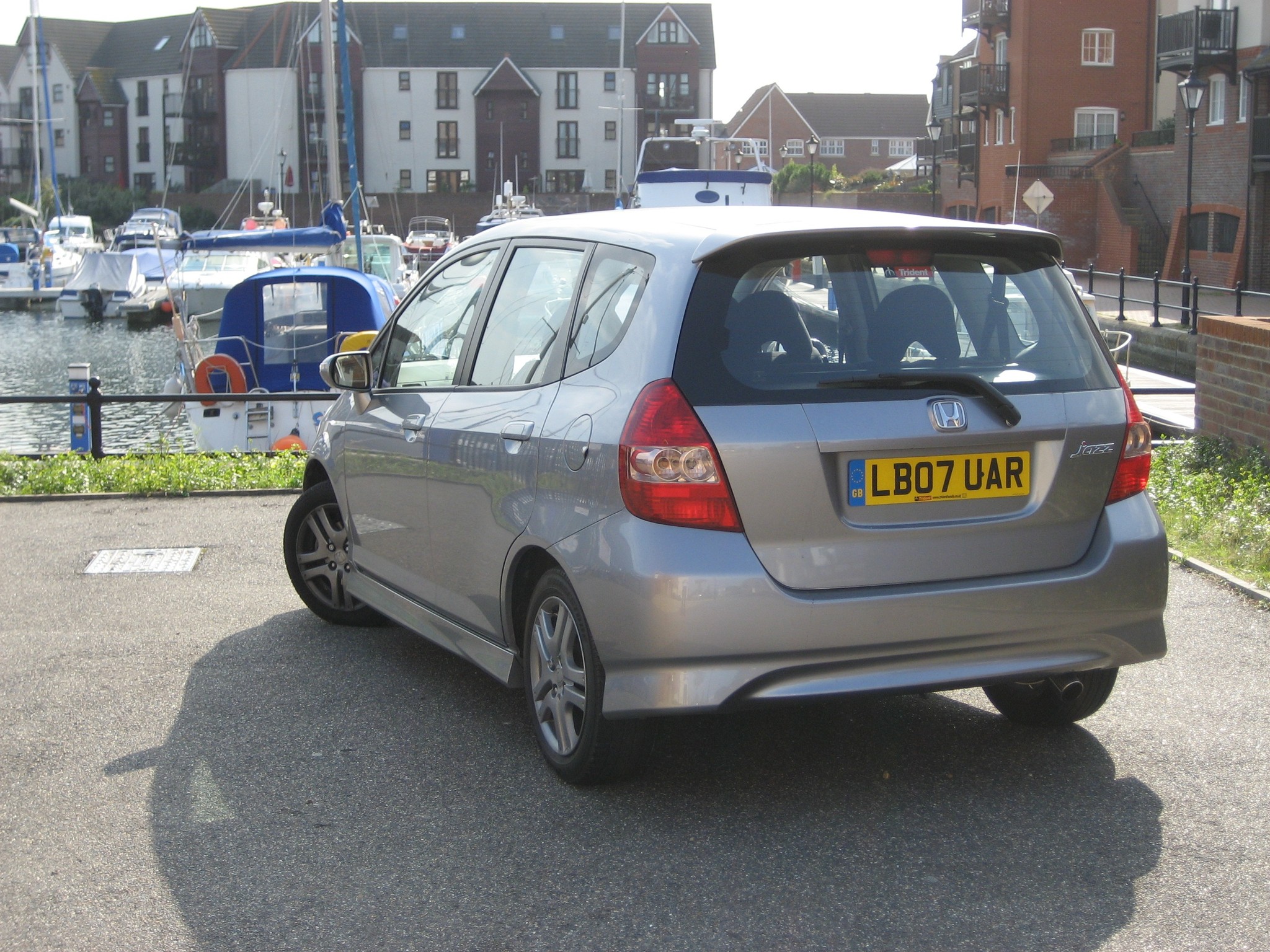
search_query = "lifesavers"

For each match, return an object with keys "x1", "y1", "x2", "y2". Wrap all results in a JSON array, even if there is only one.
[
  {"x1": 338, "y1": 330, "x2": 381, "y2": 354},
  {"x1": 194, "y1": 353, "x2": 247, "y2": 408}
]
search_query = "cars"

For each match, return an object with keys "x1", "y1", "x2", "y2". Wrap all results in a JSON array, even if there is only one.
[{"x1": 283, "y1": 204, "x2": 1172, "y2": 786}]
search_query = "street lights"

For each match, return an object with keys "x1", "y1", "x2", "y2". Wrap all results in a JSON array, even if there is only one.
[
  {"x1": 805, "y1": 134, "x2": 819, "y2": 207},
  {"x1": 779, "y1": 144, "x2": 788, "y2": 168},
  {"x1": 924, "y1": 112, "x2": 944, "y2": 217},
  {"x1": 1177, "y1": 62, "x2": 1207, "y2": 325},
  {"x1": 733, "y1": 148, "x2": 744, "y2": 170}
]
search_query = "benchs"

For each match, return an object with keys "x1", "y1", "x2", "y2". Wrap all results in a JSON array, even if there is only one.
[{"x1": 724, "y1": 286, "x2": 1018, "y2": 381}]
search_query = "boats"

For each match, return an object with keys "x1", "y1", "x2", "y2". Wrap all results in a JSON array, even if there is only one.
[
  {"x1": 1, "y1": 1, "x2": 549, "y2": 336},
  {"x1": 151, "y1": 222, "x2": 400, "y2": 453}
]
